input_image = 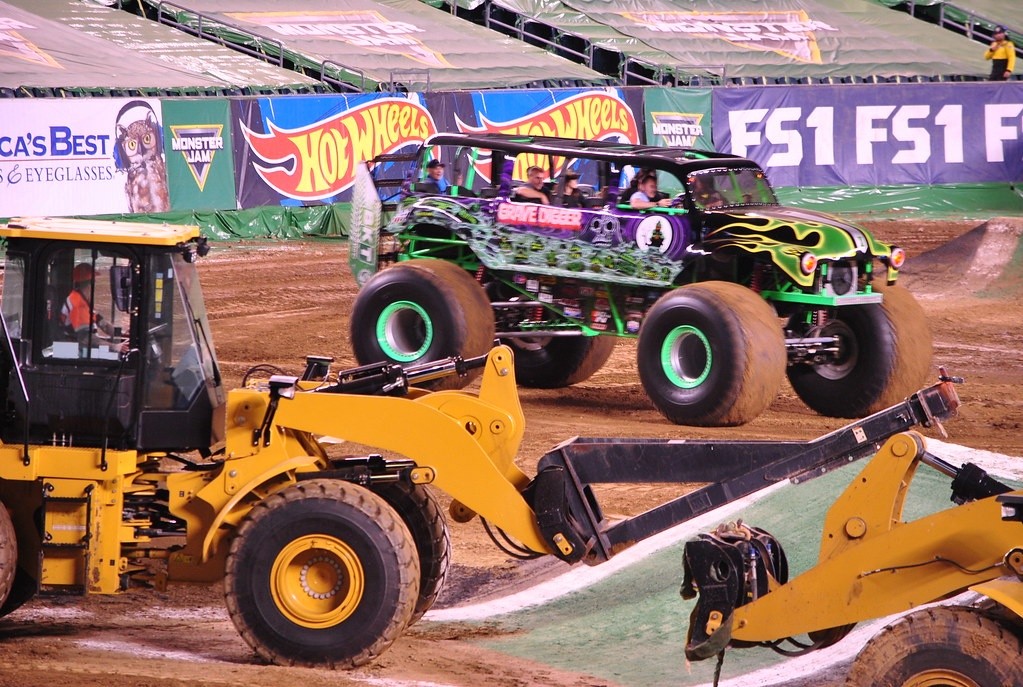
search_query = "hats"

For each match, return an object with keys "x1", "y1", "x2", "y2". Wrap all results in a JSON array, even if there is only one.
[
  {"x1": 994, "y1": 25, "x2": 1007, "y2": 33},
  {"x1": 74, "y1": 263, "x2": 101, "y2": 282},
  {"x1": 564, "y1": 169, "x2": 581, "y2": 180},
  {"x1": 427, "y1": 159, "x2": 445, "y2": 168}
]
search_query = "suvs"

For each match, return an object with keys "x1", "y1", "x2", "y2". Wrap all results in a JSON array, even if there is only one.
[{"x1": 344, "y1": 128, "x2": 935, "y2": 428}]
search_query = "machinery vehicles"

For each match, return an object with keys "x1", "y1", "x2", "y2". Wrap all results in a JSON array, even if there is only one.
[
  {"x1": 0, "y1": 209, "x2": 961, "y2": 674},
  {"x1": 676, "y1": 430, "x2": 1023, "y2": 687}
]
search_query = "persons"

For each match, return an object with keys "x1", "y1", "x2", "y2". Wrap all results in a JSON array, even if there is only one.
[
  {"x1": 515, "y1": 166, "x2": 724, "y2": 211},
  {"x1": 423, "y1": 159, "x2": 451, "y2": 194},
  {"x1": 984, "y1": 26, "x2": 1016, "y2": 81},
  {"x1": 61, "y1": 263, "x2": 132, "y2": 354}
]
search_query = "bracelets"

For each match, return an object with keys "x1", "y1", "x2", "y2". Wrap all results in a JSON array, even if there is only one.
[{"x1": 656, "y1": 201, "x2": 659, "y2": 206}]
